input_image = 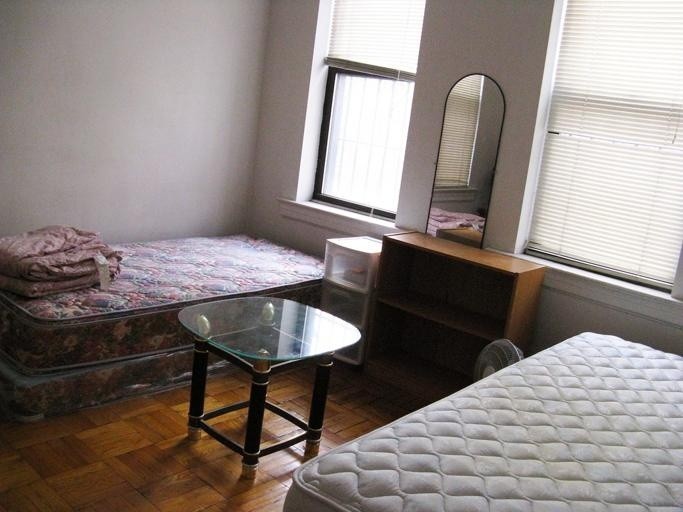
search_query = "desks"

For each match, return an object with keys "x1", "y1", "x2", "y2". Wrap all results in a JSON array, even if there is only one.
[{"x1": 177, "y1": 296, "x2": 362, "y2": 481}]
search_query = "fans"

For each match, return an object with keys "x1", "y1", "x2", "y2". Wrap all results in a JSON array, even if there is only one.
[{"x1": 473, "y1": 338, "x2": 524, "y2": 381}]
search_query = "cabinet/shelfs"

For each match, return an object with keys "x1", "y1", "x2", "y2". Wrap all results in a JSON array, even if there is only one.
[
  {"x1": 321, "y1": 234, "x2": 382, "y2": 365},
  {"x1": 363, "y1": 230, "x2": 547, "y2": 404},
  {"x1": 436, "y1": 226, "x2": 482, "y2": 248}
]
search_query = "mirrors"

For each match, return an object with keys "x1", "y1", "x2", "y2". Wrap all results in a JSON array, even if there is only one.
[{"x1": 424, "y1": 72, "x2": 506, "y2": 248}]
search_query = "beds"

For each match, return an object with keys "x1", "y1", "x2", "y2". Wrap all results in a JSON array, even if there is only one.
[
  {"x1": 0, "y1": 232, "x2": 325, "y2": 423},
  {"x1": 282, "y1": 330, "x2": 683, "y2": 512},
  {"x1": 426, "y1": 208, "x2": 485, "y2": 238}
]
